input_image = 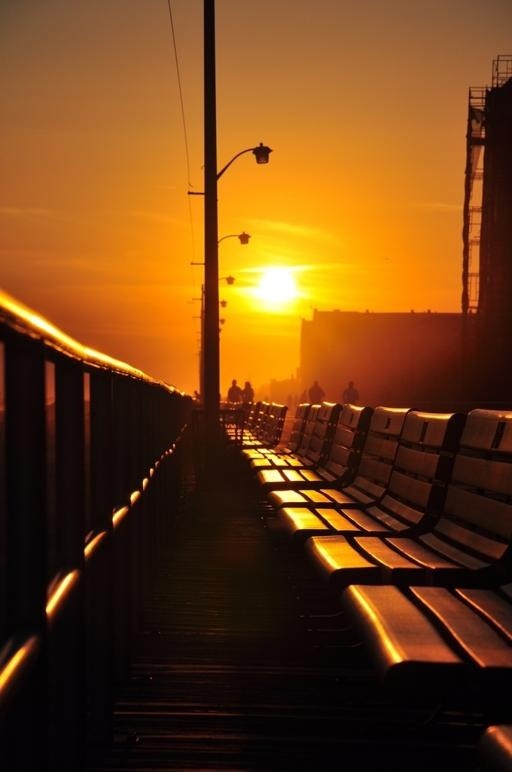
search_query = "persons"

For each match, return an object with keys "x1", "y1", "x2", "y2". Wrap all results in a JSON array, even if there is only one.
[
  {"x1": 342, "y1": 381, "x2": 358, "y2": 402},
  {"x1": 309, "y1": 380, "x2": 326, "y2": 401},
  {"x1": 241, "y1": 381, "x2": 255, "y2": 404},
  {"x1": 227, "y1": 379, "x2": 243, "y2": 402}
]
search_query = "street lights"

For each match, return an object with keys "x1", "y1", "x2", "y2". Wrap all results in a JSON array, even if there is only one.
[{"x1": 188, "y1": 142, "x2": 272, "y2": 523}]
